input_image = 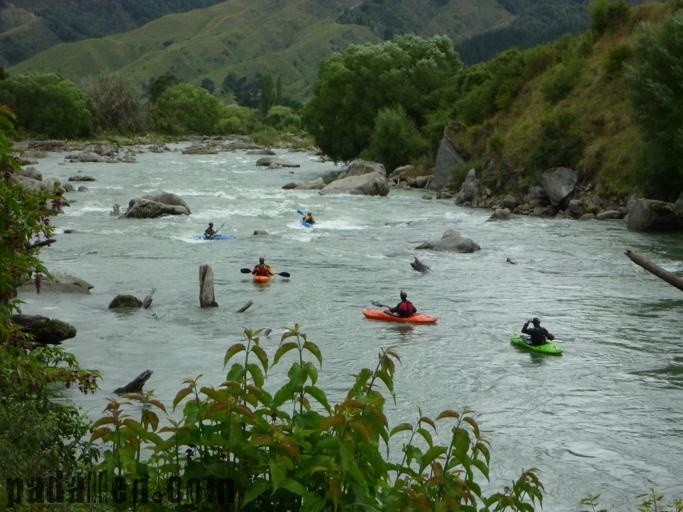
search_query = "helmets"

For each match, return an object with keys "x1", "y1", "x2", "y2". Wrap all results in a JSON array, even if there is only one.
[
  {"x1": 260, "y1": 258, "x2": 264, "y2": 262},
  {"x1": 307, "y1": 212, "x2": 312, "y2": 215},
  {"x1": 400, "y1": 291, "x2": 407, "y2": 298},
  {"x1": 209, "y1": 223, "x2": 213, "y2": 226},
  {"x1": 532, "y1": 318, "x2": 540, "y2": 324}
]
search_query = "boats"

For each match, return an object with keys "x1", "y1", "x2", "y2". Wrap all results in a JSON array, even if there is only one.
[
  {"x1": 301, "y1": 219, "x2": 311, "y2": 227},
  {"x1": 193, "y1": 234, "x2": 233, "y2": 240},
  {"x1": 253, "y1": 267, "x2": 271, "y2": 283},
  {"x1": 511, "y1": 335, "x2": 562, "y2": 355},
  {"x1": 362, "y1": 308, "x2": 436, "y2": 325}
]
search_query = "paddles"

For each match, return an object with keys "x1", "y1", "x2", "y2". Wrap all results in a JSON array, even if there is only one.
[
  {"x1": 296, "y1": 210, "x2": 304, "y2": 216},
  {"x1": 371, "y1": 300, "x2": 390, "y2": 308},
  {"x1": 240, "y1": 268, "x2": 291, "y2": 278}
]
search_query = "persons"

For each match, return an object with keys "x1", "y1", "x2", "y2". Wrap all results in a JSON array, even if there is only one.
[
  {"x1": 302, "y1": 212, "x2": 315, "y2": 224},
  {"x1": 205, "y1": 223, "x2": 216, "y2": 236},
  {"x1": 522, "y1": 317, "x2": 555, "y2": 346},
  {"x1": 388, "y1": 291, "x2": 417, "y2": 317},
  {"x1": 252, "y1": 256, "x2": 274, "y2": 277}
]
